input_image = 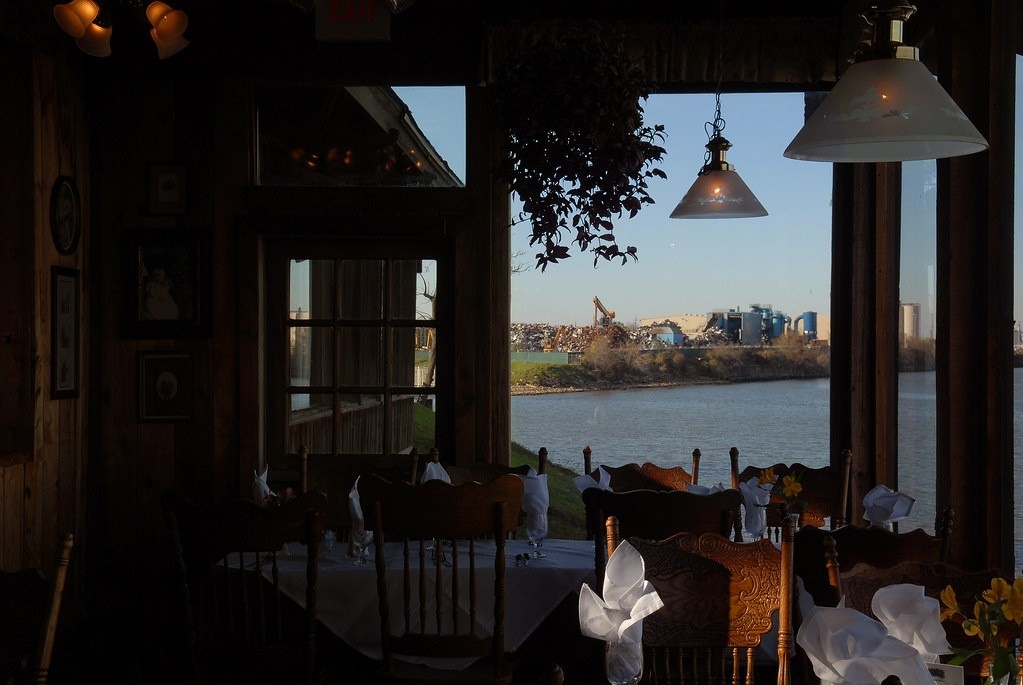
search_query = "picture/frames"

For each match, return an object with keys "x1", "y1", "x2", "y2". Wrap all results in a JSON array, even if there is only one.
[
  {"x1": 50, "y1": 264, "x2": 81, "y2": 400},
  {"x1": 136, "y1": 235, "x2": 210, "y2": 336},
  {"x1": 136, "y1": 350, "x2": 194, "y2": 424},
  {"x1": 50, "y1": 176, "x2": 83, "y2": 255},
  {"x1": 148, "y1": 161, "x2": 189, "y2": 216}
]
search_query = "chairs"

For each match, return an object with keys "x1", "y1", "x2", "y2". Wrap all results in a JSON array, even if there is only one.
[
  {"x1": 161, "y1": 444, "x2": 1015, "y2": 685},
  {"x1": 0, "y1": 532, "x2": 75, "y2": 685}
]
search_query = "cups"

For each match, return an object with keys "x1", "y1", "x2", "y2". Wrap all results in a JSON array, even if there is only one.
[
  {"x1": 605, "y1": 640, "x2": 644, "y2": 685},
  {"x1": 869, "y1": 510, "x2": 891, "y2": 532},
  {"x1": 320, "y1": 529, "x2": 337, "y2": 559}
]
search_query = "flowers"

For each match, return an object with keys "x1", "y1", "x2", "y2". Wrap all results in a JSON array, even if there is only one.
[
  {"x1": 752, "y1": 464, "x2": 811, "y2": 514},
  {"x1": 937, "y1": 577, "x2": 1023, "y2": 685}
]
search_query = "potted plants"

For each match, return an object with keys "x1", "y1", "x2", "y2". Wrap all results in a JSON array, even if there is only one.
[{"x1": 486, "y1": 2, "x2": 666, "y2": 268}]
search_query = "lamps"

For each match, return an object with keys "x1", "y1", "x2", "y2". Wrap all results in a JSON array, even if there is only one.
[
  {"x1": 780, "y1": 0, "x2": 990, "y2": 163},
  {"x1": 667, "y1": 0, "x2": 768, "y2": 220},
  {"x1": 53, "y1": 0, "x2": 190, "y2": 60}
]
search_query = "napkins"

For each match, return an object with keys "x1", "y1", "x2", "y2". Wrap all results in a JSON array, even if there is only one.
[
  {"x1": 578, "y1": 539, "x2": 665, "y2": 680},
  {"x1": 871, "y1": 583, "x2": 955, "y2": 662},
  {"x1": 797, "y1": 579, "x2": 935, "y2": 685},
  {"x1": 862, "y1": 484, "x2": 916, "y2": 532},
  {"x1": 346, "y1": 474, "x2": 373, "y2": 543},
  {"x1": 739, "y1": 475, "x2": 779, "y2": 534},
  {"x1": 572, "y1": 464, "x2": 614, "y2": 494},
  {"x1": 683, "y1": 479, "x2": 724, "y2": 494},
  {"x1": 421, "y1": 462, "x2": 452, "y2": 484},
  {"x1": 251, "y1": 462, "x2": 277, "y2": 505},
  {"x1": 519, "y1": 467, "x2": 549, "y2": 539}
]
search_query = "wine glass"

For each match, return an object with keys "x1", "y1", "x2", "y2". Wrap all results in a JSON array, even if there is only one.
[
  {"x1": 351, "y1": 519, "x2": 373, "y2": 566},
  {"x1": 526, "y1": 513, "x2": 549, "y2": 558},
  {"x1": 745, "y1": 505, "x2": 766, "y2": 542}
]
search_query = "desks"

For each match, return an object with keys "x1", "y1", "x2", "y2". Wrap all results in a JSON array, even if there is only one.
[{"x1": 202, "y1": 537, "x2": 816, "y2": 672}]
